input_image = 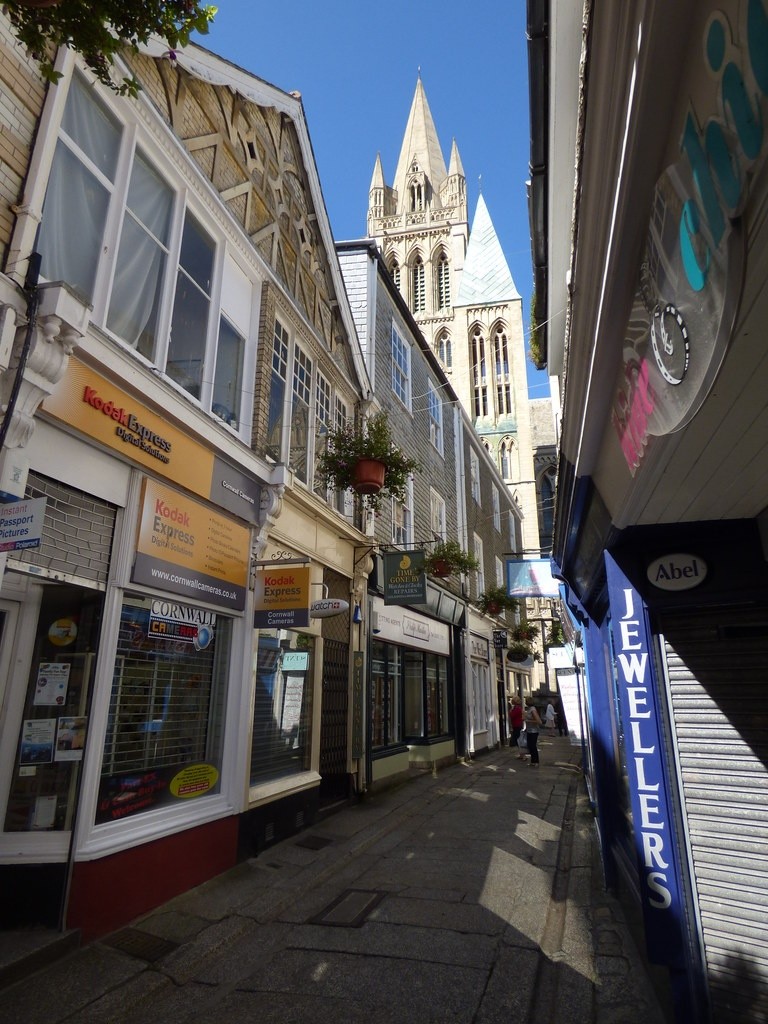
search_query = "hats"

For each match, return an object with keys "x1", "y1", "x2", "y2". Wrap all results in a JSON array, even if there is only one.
[
  {"x1": 513, "y1": 696, "x2": 522, "y2": 705},
  {"x1": 526, "y1": 695, "x2": 533, "y2": 704}
]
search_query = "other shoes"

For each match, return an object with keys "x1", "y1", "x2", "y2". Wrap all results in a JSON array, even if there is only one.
[
  {"x1": 517, "y1": 754, "x2": 525, "y2": 759},
  {"x1": 534, "y1": 764, "x2": 539, "y2": 768},
  {"x1": 565, "y1": 734, "x2": 568, "y2": 736},
  {"x1": 548, "y1": 734, "x2": 556, "y2": 737},
  {"x1": 560, "y1": 733, "x2": 562, "y2": 736},
  {"x1": 527, "y1": 763, "x2": 535, "y2": 766},
  {"x1": 523, "y1": 755, "x2": 528, "y2": 761}
]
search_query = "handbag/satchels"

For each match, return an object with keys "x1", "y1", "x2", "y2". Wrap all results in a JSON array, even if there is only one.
[{"x1": 517, "y1": 730, "x2": 527, "y2": 747}]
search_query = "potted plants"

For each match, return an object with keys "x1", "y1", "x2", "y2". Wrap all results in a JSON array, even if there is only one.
[
  {"x1": 309, "y1": 404, "x2": 423, "y2": 520},
  {"x1": 475, "y1": 580, "x2": 523, "y2": 619},
  {"x1": 506, "y1": 621, "x2": 541, "y2": 644},
  {"x1": 411, "y1": 540, "x2": 483, "y2": 579}
]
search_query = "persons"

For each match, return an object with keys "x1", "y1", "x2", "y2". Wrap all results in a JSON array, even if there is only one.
[
  {"x1": 545, "y1": 695, "x2": 567, "y2": 736},
  {"x1": 507, "y1": 696, "x2": 527, "y2": 761},
  {"x1": 520, "y1": 696, "x2": 542, "y2": 767}
]
